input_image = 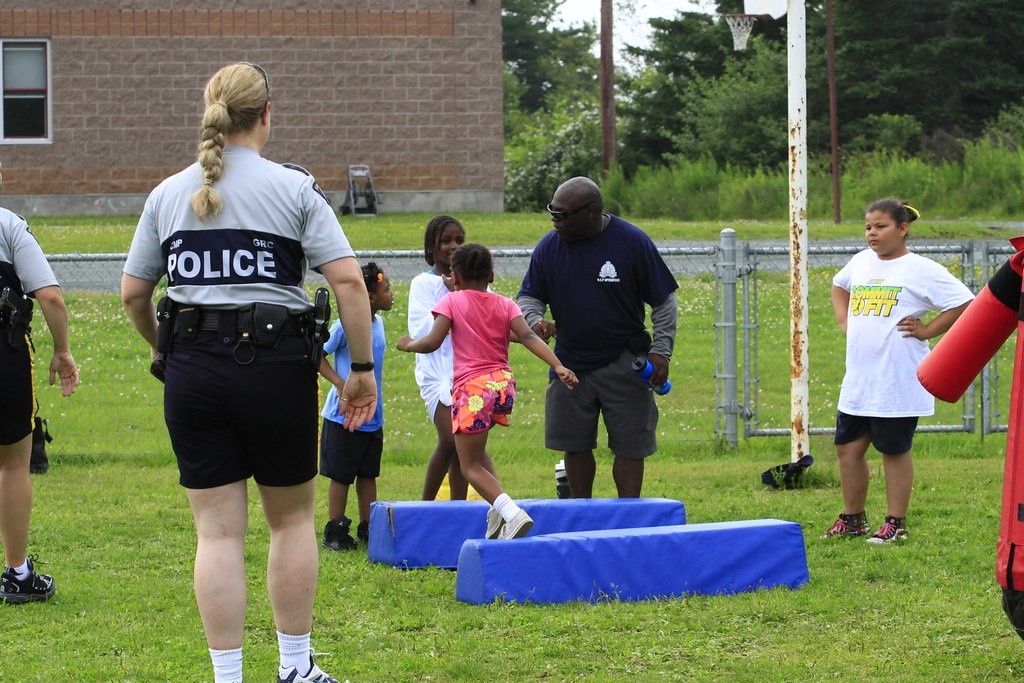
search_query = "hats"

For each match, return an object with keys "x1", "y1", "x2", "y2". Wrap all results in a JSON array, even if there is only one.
[{"x1": 760, "y1": 454, "x2": 815, "y2": 489}]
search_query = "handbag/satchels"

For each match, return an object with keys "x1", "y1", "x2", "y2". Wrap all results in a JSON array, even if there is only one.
[{"x1": 29, "y1": 416, "x2": 52, "y2": 474}]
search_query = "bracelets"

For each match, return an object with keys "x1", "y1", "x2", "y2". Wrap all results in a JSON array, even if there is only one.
[{"x1": 350, "y1": 362, "x2": 375, "y2": 372}]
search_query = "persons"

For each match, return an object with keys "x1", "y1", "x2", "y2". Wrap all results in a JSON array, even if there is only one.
[
  {"x1": 514, "y1": 176, "x2": 680, "y2": 500},
  {"x1": 818, "y1": 198, "x2": 976, "y2": 545},
  {"x1": 397, "y1": 243, "x2": 580, "y2": 540},
  {"x1": 0, "y1": 208, "x2": 78, "y2": 605},
  {"x1": 317, "y1": 261, "x2": 395, "y2": 552},
  {"x1": 407, "y1": 214, "x2": 555, "y2": 503},
  {"x1": 121, "y1": 62, "x2": 377, "y2": 683}
]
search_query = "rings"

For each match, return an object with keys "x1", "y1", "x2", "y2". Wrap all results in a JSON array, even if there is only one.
[
  {"x1": 341, "y1": 398, "x2": 349, "y2": 402},
  {"x1": 543, "y1": 328, "x2": 547, "y2": 333}
]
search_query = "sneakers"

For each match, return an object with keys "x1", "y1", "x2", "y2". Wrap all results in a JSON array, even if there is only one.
[
  {"x1": 818, "y1": 509, "x2": 872, "y2": 541},
  {"x1": 865, "y1": 515, "x2": 908, "y2": 546},
  {"x1": 484, "y1": 509, "x2": 506, "y2": 539},
  {"x1": 321, "y1": 516, "x2": 358, "y2": 553},
  {"x1": 356, "y1": 520, "x2": 370, "y2": 545},
  {"x1": 496, "y1": 509, "x2": 534, "y2": 540},
  {"x1": 277, "y1": 648, "x2": 341, "y2": 683},
  {"x1": 0, "y1": 554, "x2": 56, "y2": 605}
]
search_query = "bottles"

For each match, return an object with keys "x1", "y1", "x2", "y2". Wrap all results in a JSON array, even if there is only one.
[
  {"x1": 555, "y1": 460, "x2": 571, "y2": 499},
  {"x1": 632, "y1": 357, "x2": 671, "y2": 396}
]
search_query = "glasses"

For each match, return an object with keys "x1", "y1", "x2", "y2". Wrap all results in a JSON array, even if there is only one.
[
  {"x1": 237, "y1": 61, "x2": 269, "y2": 112},
  {"x1": 546, "y1": 200, "x2": 603, "y2": 222}
]
